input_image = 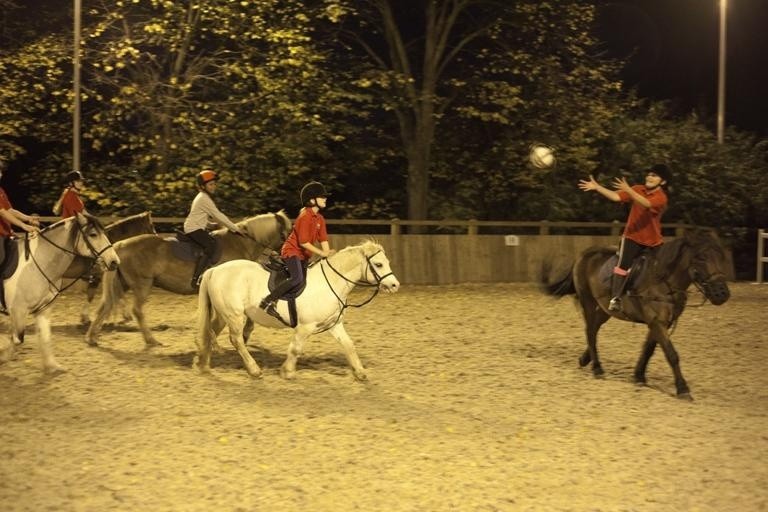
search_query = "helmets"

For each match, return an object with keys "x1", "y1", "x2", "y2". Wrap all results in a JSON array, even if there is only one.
[
  {"x1": 301, "y1": 182, "x2": 332, "y2": 206},
  {"x1": 197, "y1": 170, "x2": 221, "y2": 185},
  {"x1": 643, "y1": 163, "x2": 672, "y2": 183},
  {"x1": 66, "y1": 171, "x2": 88, "y2": 183}
]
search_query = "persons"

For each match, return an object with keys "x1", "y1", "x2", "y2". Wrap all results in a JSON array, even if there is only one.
[
  {"x1": 0, "y1": 172, "x2": 41, "y2": 315},
  {"x1": 181, "y1": 169, "x2": 244, "y2": 289},
  {"x1": 51, "y1": 171, "x2": 92, "y2": 219},
  {"x1": 258, "y1": 178, "x2": 334, "y2": 319},
  {"x1": 576, "y1": 163, "x2": 674, "y2": 313}
]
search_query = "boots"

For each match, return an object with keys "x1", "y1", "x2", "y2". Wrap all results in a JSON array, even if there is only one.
[
  {"x1": 609, "y1": 266, "x2": 629, "y2": 312},
  {"x1": 260, "y1": 295, "x2": 280, "y2": 318}
]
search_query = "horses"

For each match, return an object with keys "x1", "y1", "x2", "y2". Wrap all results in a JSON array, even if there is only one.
[
  {"x1": 0, "y1": 207, "x2": 121, "y2": 376},
  {"x1": 82, "y1": 208, "x2": 293, "y2": 346},
  {"x1": 191, "y1": 234, "x2": 401, "y2": 381},
  {"x1": 61, "y1": 210, "x2": 159, "y2": 327},
  {"x1": 536, "y1": 226, "x2": 731, "y2": 402}
]
